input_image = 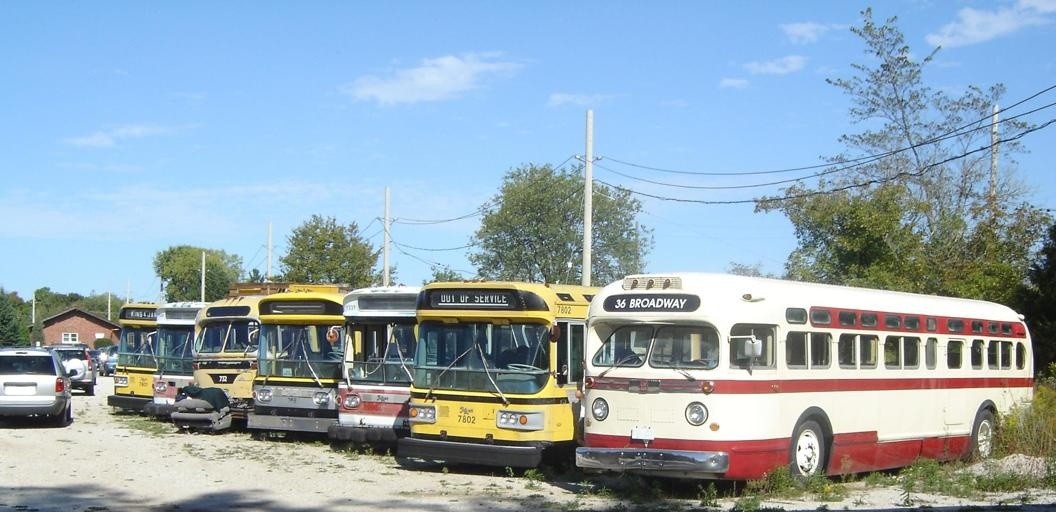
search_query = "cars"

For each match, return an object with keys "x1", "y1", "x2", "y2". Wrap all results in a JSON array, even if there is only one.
[
  {"x1": 74, "y1": 341, "x2": 122, "y2": 379},
  {"x1": 0, "y1": 346, "x2": 78, "y2": 426}
]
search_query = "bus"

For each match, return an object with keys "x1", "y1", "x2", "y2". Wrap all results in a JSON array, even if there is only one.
[
  {"x1": 327, "y1": 285, "x2": 488, "y2": 442},
  {"x1": 248, "y1": 284, "x2": 396, "y2": 434},
  {"x1": 168, "y1": 281, "x2": 320, "y2": 434},
  {"x1": 143, "y1": 300, "x2": 213, "y2": 418},
  {"x1": 109, "y1": 301, "x2": 158, "y2": 409},
  {"x1": 407, "y1": 280, "x2": 876, "y2": 469},
  {"x1": 575, "y1": 268, "x2": 1037, "y2": 489}
]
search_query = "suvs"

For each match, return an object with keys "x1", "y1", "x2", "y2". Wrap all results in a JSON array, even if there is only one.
[{"x1": 46, "y1": 342, "x2": 100, "y2": 397}]
